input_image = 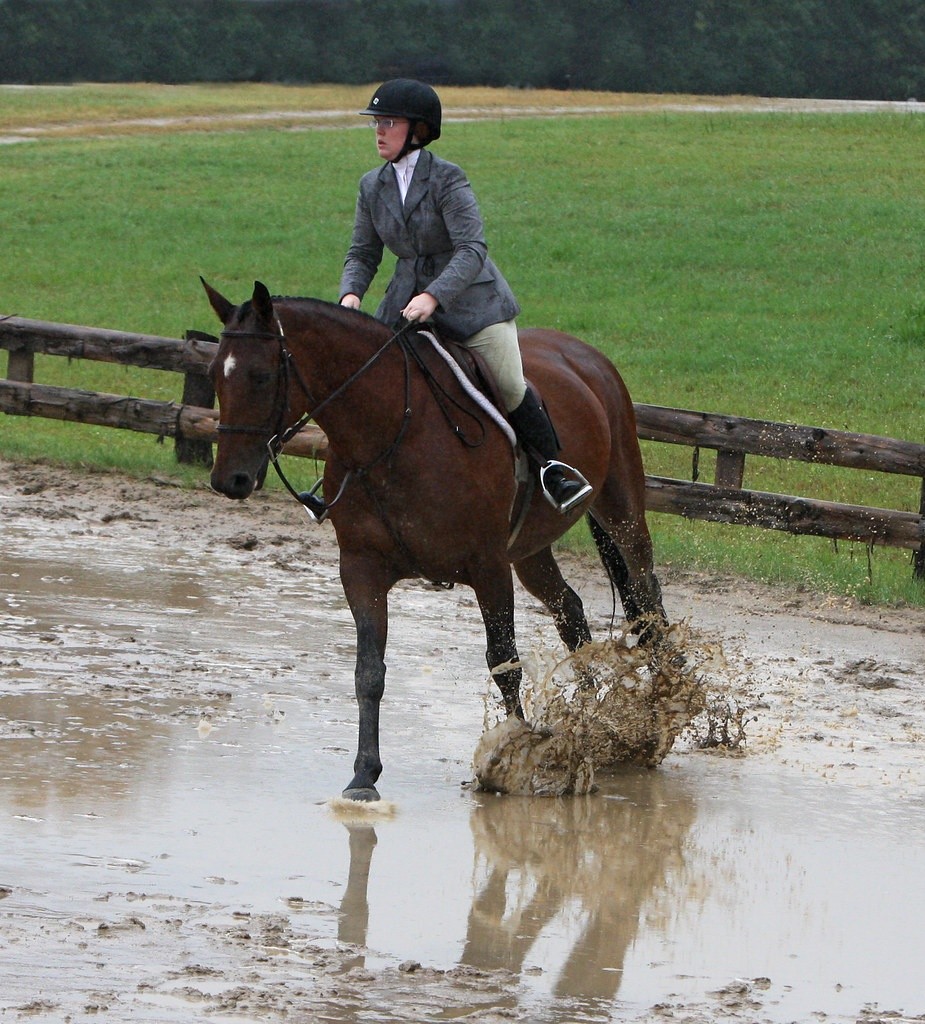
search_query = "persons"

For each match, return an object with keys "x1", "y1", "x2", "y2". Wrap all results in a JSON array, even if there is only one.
[{"x1": 298, "y1": 80, "x2": 580, "y2": 519}]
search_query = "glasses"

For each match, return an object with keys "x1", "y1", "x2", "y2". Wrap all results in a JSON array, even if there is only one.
[{"x1": 367, "y1": 119, "x2": 411, "y2": 127}]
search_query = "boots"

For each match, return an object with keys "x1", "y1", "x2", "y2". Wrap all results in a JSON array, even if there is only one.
[
  {"x1": 509, "y1": 387, "x2": 582, "y2": 503},
  {"x1": 299, "y1": 490, "x2": 326, "y2": 513}
]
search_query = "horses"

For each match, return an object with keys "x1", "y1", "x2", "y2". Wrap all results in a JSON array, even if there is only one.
[{"x1": 195, "y1": 272, "x2": 681, "y2": 803}]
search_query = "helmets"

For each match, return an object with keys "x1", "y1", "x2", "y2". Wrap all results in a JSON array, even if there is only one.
[{"x1": 359, "y1": 78, "x2": 441, "y2": 141}]
